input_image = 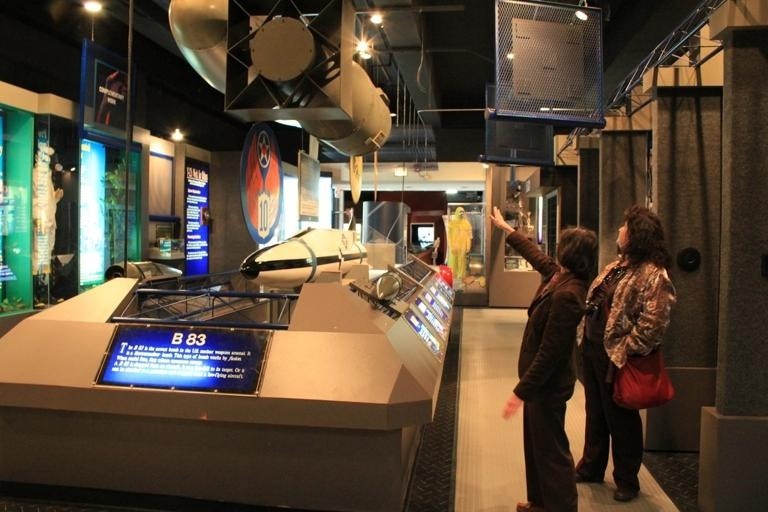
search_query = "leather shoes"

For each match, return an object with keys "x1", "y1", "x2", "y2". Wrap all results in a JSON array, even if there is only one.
[
  {"x1": 517, "y1": 501, "x2": 542, "y2": 512},
  {"x1": 574, "y1": 473, "x2": 605, "y2": 485},
  {"x1": 613, "y1": 487, "x2": 638, "y2": 502}
]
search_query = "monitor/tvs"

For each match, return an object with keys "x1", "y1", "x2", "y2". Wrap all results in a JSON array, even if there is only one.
[
  {"x1": 403, "y1": 274, "x2": 455, "y2": 362},
  {"x1": 98, "y1": 323, "x2": 273, "y2": 396}
]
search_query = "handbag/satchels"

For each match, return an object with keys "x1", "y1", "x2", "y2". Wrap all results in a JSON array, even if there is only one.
[{"x1": 614, "y1": 352, "x2": 676, "y2": 411}]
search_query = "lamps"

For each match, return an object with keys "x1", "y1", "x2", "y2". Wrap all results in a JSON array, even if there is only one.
[{"x1": 573, "y1": 0, "x2": 588, "y2": 24}]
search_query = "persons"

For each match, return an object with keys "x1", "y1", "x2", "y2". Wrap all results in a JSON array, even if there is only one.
[
  {"x1": 445, "y1": 207, "x2": 472, "y2": 278},
  {"x1": 32, "y1": 142, "x2": 63, "y2": 308},
  {"x1": 488, "y1": 206, "x2": 596, "y2": 512},
  {"x1": 575, "y1": 206, "x2": 677, "y2": 501}
]
